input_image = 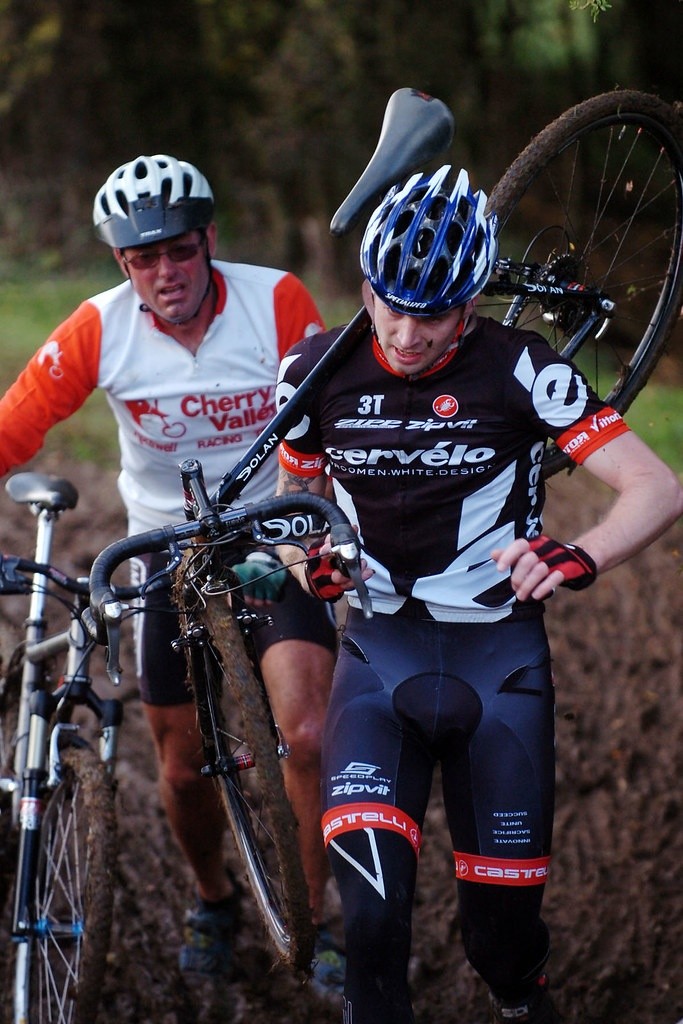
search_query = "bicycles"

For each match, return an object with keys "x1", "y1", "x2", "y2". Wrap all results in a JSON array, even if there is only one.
[
  {"x1": 1, "y1": 471, "x2": 179, "y2": 1024},
  {"x1": 88, "y1": 84, "x2": 683, "y2": 984}
]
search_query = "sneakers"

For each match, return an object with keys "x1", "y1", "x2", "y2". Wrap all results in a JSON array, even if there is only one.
[
  {"x1": 178, "y1": 870, "x2": 243, "y2": 997},
  {"x1": 304, "y1": 926, "x2": 350, "y2": 1013}
]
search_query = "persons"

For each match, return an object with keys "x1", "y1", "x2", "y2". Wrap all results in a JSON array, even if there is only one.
[
  {"x1": 273, "y1": 170, "x2": 683, "y2": 1024},
  {"x1": 0, "y1": 154, "x2": 348, "y2": 1024},
  {"x1": 0, "y1": 0, "x2": 683, "y2": 379}
]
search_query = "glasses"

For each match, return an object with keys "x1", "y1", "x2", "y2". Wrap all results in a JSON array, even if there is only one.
[{"x1": 121, "y1": 239, "x2": 207, "y2": 270}]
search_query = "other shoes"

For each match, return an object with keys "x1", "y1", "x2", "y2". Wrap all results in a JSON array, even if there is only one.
[{"x1": 488, "y1": 974, "x2": 563, "y2": 1024}]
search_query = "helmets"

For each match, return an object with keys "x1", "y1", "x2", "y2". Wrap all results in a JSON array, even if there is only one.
[
  {"x1": 359, "y1": 165, "x2": 500, "y2": 317},
  {"x1": 92, "y1": 155, "x2": 214, "y2": 247}
]
